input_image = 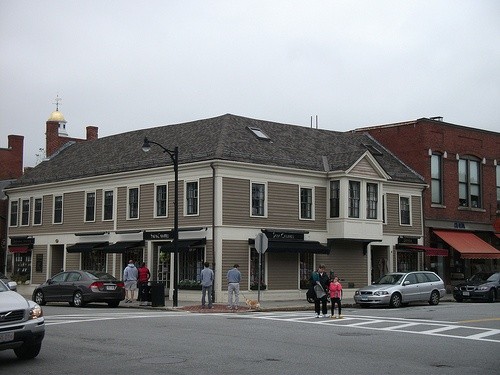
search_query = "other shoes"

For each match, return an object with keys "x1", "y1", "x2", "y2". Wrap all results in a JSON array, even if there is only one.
[
  {"x1": 324, "y1": 316, "x2": 327, "y2": 318},
  {"x1": 316, "y1": 316, "x2": 320, "y2": 318},
  {"x1": 332, "y1": 315, "x2": 336, "y2": 318},
  {"x1": 339, "y1": 316, "x2": 343, "y2": 318},
  {"x1": 226, "y1": 306, "x2": 238, "y2": 310}
]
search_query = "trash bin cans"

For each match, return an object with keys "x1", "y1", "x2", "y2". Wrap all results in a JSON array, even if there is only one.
[{"x1": 151, "y1": 282, "x2": 166, "y2": 307}]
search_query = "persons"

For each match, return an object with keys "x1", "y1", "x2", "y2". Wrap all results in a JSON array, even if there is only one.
[
  {"x1": 200, "y1": 261, "x2": 215, "y2": 310},
  {"x1": 226, "y1": 263, "x2": 243, "y2": 311},
  {"x1": 329, "y1": 276, "x2": 345, "y2": 320},
  {"x1": 311, "y1": 263, "x2": 332, "y2": 319},
  {"x1": 136, "y1": 261, "x2": 151, "y2": 301},
  {"x1": 123, "y1": 260, "x2": 138, "y2": 304}
]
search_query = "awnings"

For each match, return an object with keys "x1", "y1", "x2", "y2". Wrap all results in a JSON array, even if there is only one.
[
  {"x1": 7, "y1": 243, "x2": 34, "y2": 254},
  {"x1": 159, "y1": 237, "x2": 207, "y2": 253},
  {"x1": 264, "y1": 239, "x2": 331, "y2": 255},
  {"x1": 433, "y1": 230, "x2": 500, "y2": 260},
  {"x1": 103, "y1": 240, "x2": 145, "y2": 254},
  {"x1": 395, "y1": 242, "x2": 448, "y2": 257},
  {"x1": 66, "y1": 241, "x2": 110, "y2": 254}
]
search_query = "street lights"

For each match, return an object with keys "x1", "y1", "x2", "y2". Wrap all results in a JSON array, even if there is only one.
[{"x1": 141, "y1": 137, "x2": 179, "y2": 307}]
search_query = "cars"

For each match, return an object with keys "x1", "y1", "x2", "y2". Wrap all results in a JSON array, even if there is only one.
[
  {"x1": 0, "y1": 276, "x2": 47, "y2": 361},
  {"x1": 452, "y1": 271, "x2": 500, "y2": 304},
  {"x1": 354, "y1": 270, "x2": 445, "y2": 308},
  {"x1": 31, "y1": 269, "x2": 126, "y2": 308}
]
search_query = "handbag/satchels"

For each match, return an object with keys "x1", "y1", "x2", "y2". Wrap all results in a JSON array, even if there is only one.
[{"x1": 314, "y1": 284, "x2": 326, "y2": 298}]
